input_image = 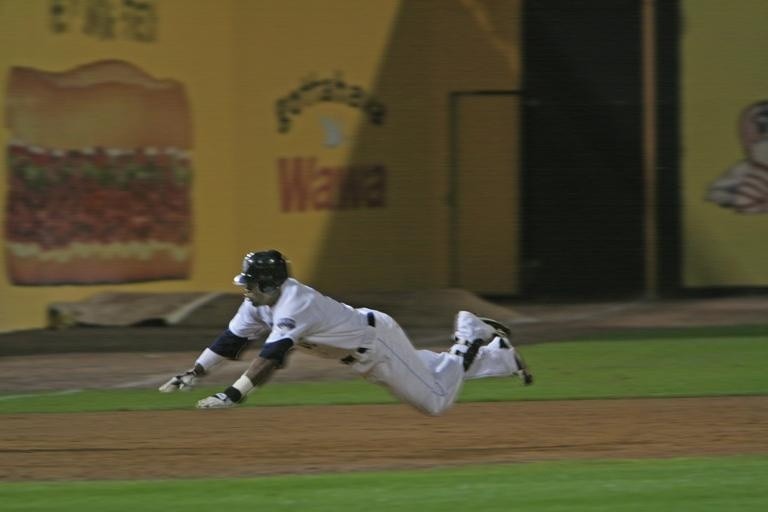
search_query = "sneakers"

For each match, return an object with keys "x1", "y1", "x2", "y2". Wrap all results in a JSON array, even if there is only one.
[
  {"x1": 498, "y1": 337, "x2": 532, "y2": 384},
  {"x1": 478, "y1": 318, "x2": 510, "y2": 337}
]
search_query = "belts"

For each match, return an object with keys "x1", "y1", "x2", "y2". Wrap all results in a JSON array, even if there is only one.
[{"x1": 341, "y1": 312, "x2": 374, "y2": 365}]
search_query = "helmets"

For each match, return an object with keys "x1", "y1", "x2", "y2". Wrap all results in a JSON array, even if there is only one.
[{"x1": 233, "y1": 250, "x2": 288, "y2": 292}]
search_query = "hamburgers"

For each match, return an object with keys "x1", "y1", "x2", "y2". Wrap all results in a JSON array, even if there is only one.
[{"x1": 7, "y1": 59, "x2": 192, "y2": 285}]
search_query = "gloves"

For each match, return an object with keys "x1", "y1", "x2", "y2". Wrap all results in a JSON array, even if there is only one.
[
  {"x1": 158, "y1": 364, "x2": 206, "y2": 392},
  {"x1": 197, "y1": 386, "x2": 241, "y2": 407}
]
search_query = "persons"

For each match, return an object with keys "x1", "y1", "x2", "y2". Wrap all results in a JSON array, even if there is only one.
[{"x1": 158, "y1": 247, "x2": 533, "y2": 410}]
